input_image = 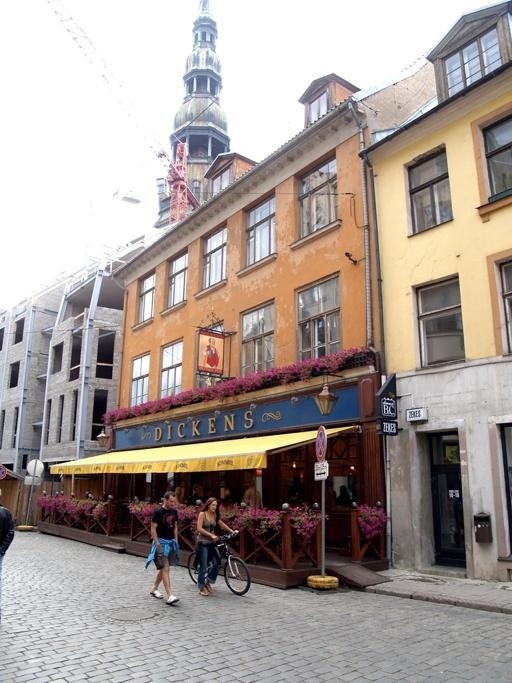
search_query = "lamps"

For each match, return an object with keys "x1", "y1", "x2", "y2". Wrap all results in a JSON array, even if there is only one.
[{"x1": 95, "y1": 423, "x2": 115, "y2": 448}]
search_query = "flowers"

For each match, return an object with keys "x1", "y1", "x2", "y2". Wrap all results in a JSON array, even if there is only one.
[
  {"x1": 357, "y1": 504, "x2": 390, "y2": 542},
  {"x1": 37, "y1": 495, "x2": 108, "y2": 523},
  {"x1": 128, "y1": 501, "x2": 329, "y2": 542},
  {"x1": 101, "y1": 346, "x2": 375, "y2": 429}
]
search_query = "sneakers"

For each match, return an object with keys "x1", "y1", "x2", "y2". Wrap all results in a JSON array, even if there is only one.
[
  {"x1": 198, "y1": 584, "x2": 213, "y2": 596},
  {"x1": 149, "y1": 589, "x2": 180, "y2": 604}
]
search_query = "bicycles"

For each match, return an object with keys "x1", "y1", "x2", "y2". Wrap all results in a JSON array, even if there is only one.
[{"x1": 187, "y1": 531, "x2": 251, "y2": 596}]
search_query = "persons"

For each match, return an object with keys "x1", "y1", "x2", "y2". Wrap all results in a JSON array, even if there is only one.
[
  {"x1": 174, "y1": 478, "x2": 186, "y2": 504},
  {"x1": 338, "y1": 484, "x2": 352, "y2": 508},
  {"x1": 196, "y1": 496, "x2": 240, "y2": 596},
  {"x1": 190, "y1": 479, "x2": 205, "y2": 500},
  {"x1": 0, "y1": 504, "x2": 15, "y2": 623},
  {"x1": 145, "y1": 489, "x2": 185, "y2": 606},
  {"x1": 242, "y1": 478, "x2": 257, "y2": 506},
  {"x1": 202, "y1": 337, "x2": 221, "y2": 370}
]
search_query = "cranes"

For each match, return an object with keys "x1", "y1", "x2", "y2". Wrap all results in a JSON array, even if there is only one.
[{"x1": 45, "y1": 0, "x2": 200, "y2": 223}]
